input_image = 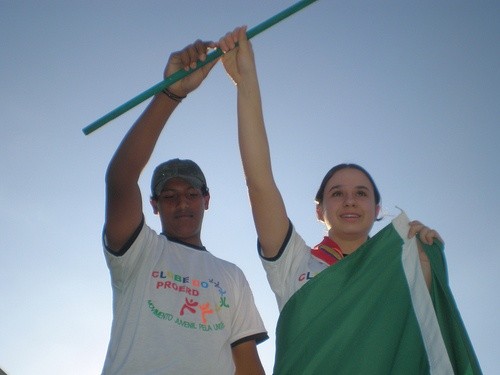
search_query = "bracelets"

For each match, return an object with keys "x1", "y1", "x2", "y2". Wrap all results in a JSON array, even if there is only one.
[{"x1": 163, "y1": 89, "x2": 185, "y2": 102}]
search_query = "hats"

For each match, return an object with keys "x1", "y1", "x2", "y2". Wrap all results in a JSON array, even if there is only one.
[{"x1": 151, "y1": 158, "x2": 209, "y2": 196}]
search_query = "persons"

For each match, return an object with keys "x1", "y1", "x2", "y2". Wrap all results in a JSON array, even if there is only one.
[
  {"x1": 217, "y1": 24, "x2": 444, "y2": 309},
  {"x1": 101, "y1": 39, "x2": 269, "y2": 375}
]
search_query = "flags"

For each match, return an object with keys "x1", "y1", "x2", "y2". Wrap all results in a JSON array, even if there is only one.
[{"x1": 273, "y1": 207, "x2": 483, "y2": 375}]
